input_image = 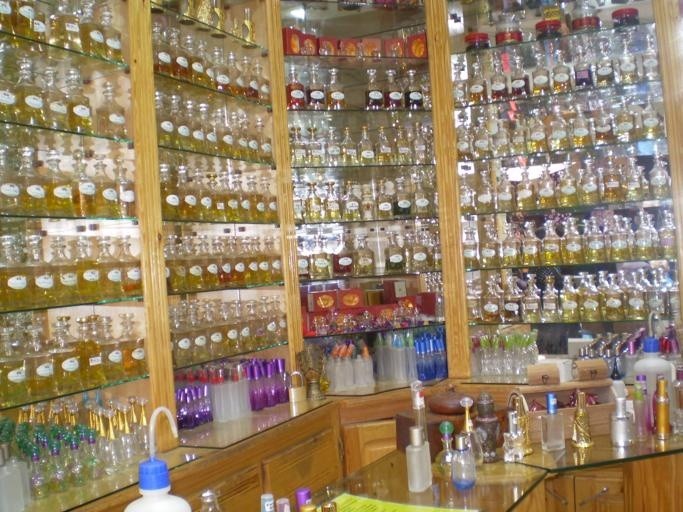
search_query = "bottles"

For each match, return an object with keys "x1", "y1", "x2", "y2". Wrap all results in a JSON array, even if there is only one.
[
  {"x1": 0, "y1": 394, "x2": 150, "y2": 501},
  {"x1": 572, "y1": 392, "x2": 594, "y2": 447},
  {"x1": 0, "y1": 0, "x2": 127, "y2": 63},
  {"x1": 0, "y1": 144, "x2": 134, "y2": 218},
  {"x1": 633, "y1": 374, "x2": 670, "y2": 440},
  {"x1": 609, "y1": 397, "x2": 634, "y2": 446},
  {"x1": 424, "y1": 272, "x2": 445, "y2": 322},
  {"x1": 150, "y1": 0, "x2": 256, "y2": 49},
  {"x1": 454, "y1": 34, "x2": 658, "y2": 106},
  {"x1": 151, "y1": 21, "x2": 271, "y2": 104},
  {"x1": 293, "y1": 174, "x2": 436, "y2": 219},
  {"x1": 541, "y1": 393, "x2": 565, "y2": 451},
  {"x1": 467, "y1": 266, "x2": 681, "y2": 323},
  {"x1": 0, "y1": 236, "x2": 143, "y2": 309},
  {"x1": 404, "y1": 69, "x2": 424, "y2": 109},
  {"x1": 308, "y1": 67, "x2": 326, "y2": 109},
  {"x1": 286, "y1": 67, "x2": 306, "y2": 109},
  {"x1": 154, "y1": 91, "x2": 271, "y2": 160},
  {"x1": 327, "y1": 68, "x2": 347, "y2": 110},
  {"x1": 0, "y1": 40, "x2": 126, "y2": 139},
  {"x1": 503, "y1": 397, "x2": 534, "y2": 463},
  {"x1": 169, "y1": 296, "x2": 287, "y2": 367},
  {"x1": 290, "y1": 120, "x2": 434, "y2": 165},
  {"x1": 459, "y1": 151, "x2": 670, "y2": 214},
  {"x1": 383, "y1": 70, "x2": 403, "y2": 109},
  {"x1": 463, "y1": 210, "x2": 675, "y2": 269},
  {"x1": 365, "y1": 70, "x2": 385, "y2": 109},
  {"x1": 159, "y1": 163, "x2": 276, "y2": 222},
  {"x1": 164, "y1": 236, "x2": 283, "y2": 292},
  {"x1": 455, "y1": 91, "x2": 665, "y2": 160},
  {"x1": 326, "y1": 328, "x2": 446, "y2": 392},
  {"x1": 470, "y1": 328, "x2": 539, "y2": 377},
  {"x1": 435, "y1": 396, "x2": 483, "y2": 490},
  {"x1": 298, "y1": 227, "x2": 441, "y2": 276},
  {"x1": 405, "y1": 426, "x2": 433, "y2": 493},
  {"x1": 174, "y1": 356, "x2": 288, "y2": 428},
  {"x1": 1, "y1": 313, "x2": 147, "y2": 405},
  {"x1": 577, "y1": 323, "x2": 678, "y2": 377}
]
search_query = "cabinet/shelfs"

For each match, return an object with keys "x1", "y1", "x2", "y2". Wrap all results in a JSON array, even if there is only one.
[
  {"x1": 0, "y1": 396, "x2": 346, "y2": 512},
  {"x1": 0, "y1": 1, "x2": 170, "y2": 452},
  {"x1": 496, "y1": 399, "x2": 683, "y2": 512},
  {"x1": 142, "y1": 0, "x2": 301, "y2": 432},
  {"x1": 274, "y1": 0, "x2": 460, "y2": 386},
  {"x1": 290, "y1": 449, "x2": 548, "y2": 512},
  {"x1": 436, "y1": 0, "x2": 683, "y2": 379},
  {"x1": 325, "y1": 379, "x2": 636, "y2": 496}
]
